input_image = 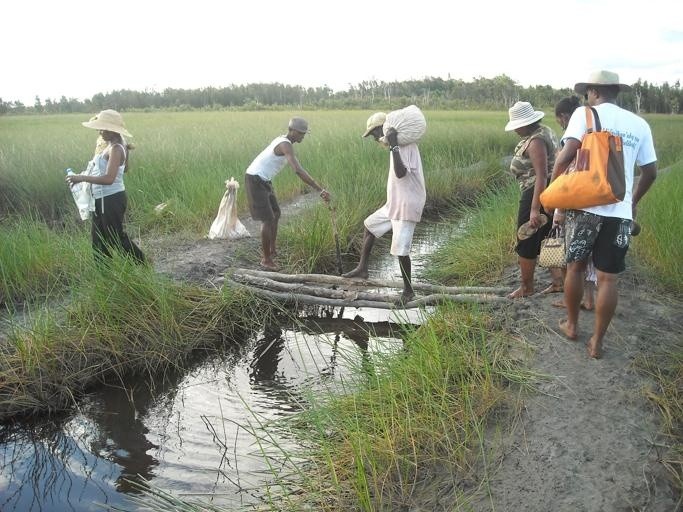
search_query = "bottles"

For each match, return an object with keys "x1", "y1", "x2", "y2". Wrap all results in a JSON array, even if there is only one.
[{"x1": 66, "y1": 168, "x2": 89, "y2": 211}]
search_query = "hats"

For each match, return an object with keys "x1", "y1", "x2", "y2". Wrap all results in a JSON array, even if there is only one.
[
  {"x1": 361, "y1": 112, "x2": 387, "y2": 137},
  {"x1": 81, "y1": 109, "x2": 132, "y2": 137},
  {"x1": 504, "y1": 101, "x2": 544, "y2": 131},
  {"x1": 288, "y1": 117, "x2": 310, "y2": 133},
  {"x1": 573, "y1": 71, "x2": 632, "y2": 95}
]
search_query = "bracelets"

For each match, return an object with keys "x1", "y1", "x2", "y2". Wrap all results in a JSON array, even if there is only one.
[{"x1": 319, "y1": 189, "x2": 325, "y2": 196}]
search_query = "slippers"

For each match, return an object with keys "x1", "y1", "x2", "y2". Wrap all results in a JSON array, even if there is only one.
[{"x1": 517, "y1": 214, "x2": 547, "y2": 240}]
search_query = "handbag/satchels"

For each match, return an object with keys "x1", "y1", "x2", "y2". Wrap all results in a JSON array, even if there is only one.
[
  {"x1": 538, "y1": 224, "x2": 566, "y2": 269},
  {"x1": 538, "y1": 106, "x2": 624, "y2": 208}
]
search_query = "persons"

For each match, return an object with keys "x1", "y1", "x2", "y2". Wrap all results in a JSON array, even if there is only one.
[
  {"x1": 65, "y1": 110, "x2": 146, "y2": 266},
  {"x1": 548, "y1": 70, "x2": 657, "y2": 357},
  {"x1": 505, "y1": 102, "x2": 565, "y2": 299},
  {"x1": 551, "y1": 96, "x2": 599, "y2": 310},
  {"x1": 245, "y1": 116, "x2": 330, "y2": 271},
  {"x1": 341, "y1": 112, "x2": 426, "y2": 306}
]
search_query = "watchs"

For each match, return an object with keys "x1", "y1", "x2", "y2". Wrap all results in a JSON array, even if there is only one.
[{"x1": 389, "y1": 145, "x2": 401, "y2": 152}]
